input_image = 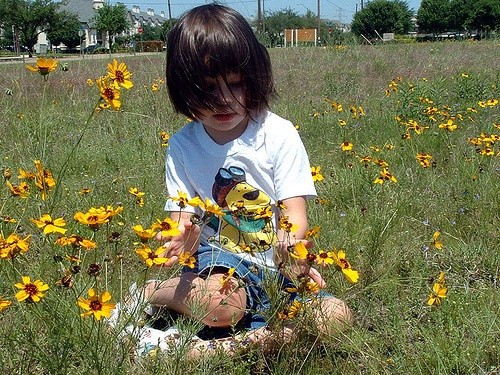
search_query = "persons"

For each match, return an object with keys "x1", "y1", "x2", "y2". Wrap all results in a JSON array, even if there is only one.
[{"x1": 106, "y1": 5, "x2": 353, "y2": 362}]
